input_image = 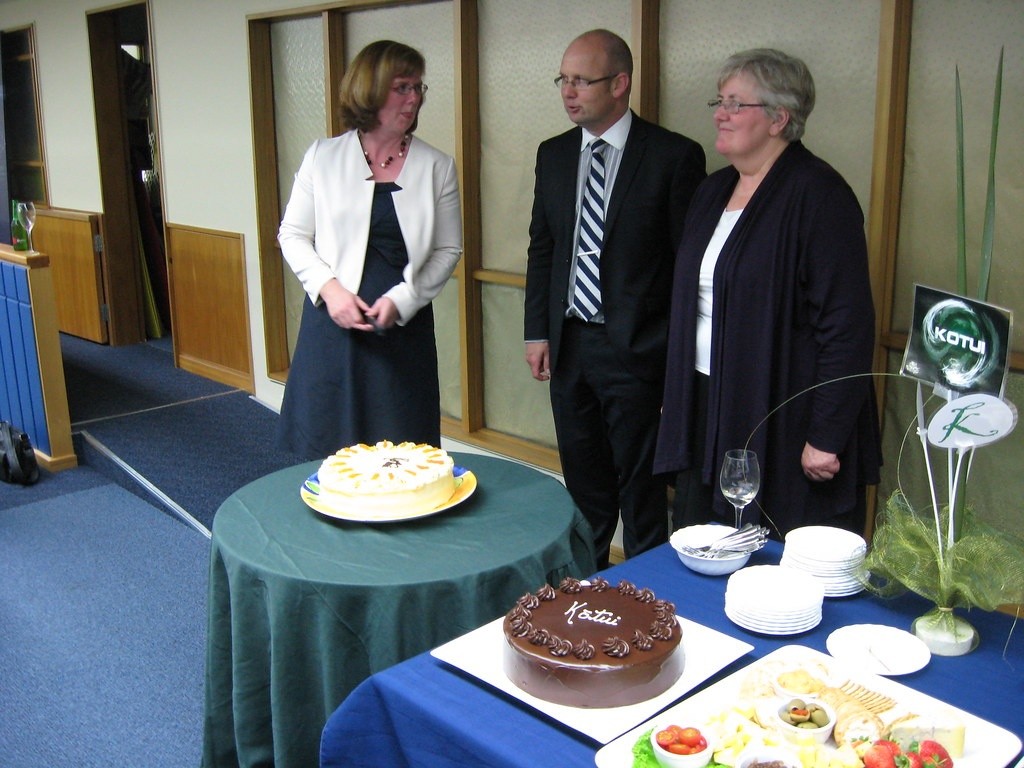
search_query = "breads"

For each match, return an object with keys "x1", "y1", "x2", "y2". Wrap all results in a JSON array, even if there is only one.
[
  {"x1": 888, "y1": 712, "x2": 965, "y2": 760},
  {"x1": 743, "y1": 661, "x2": 779, "y2": 697}
]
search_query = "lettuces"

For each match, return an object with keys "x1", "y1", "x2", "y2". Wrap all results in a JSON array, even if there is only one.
[{"x1": 632, "y1": 728, "x2": 734, "y2": 767}]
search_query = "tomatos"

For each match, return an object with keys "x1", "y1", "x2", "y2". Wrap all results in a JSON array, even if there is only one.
[{"x1": 656, "y1": 724, "x2": 706, "y2": 756}]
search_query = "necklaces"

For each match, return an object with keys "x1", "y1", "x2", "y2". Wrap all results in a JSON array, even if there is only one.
[{"x1": 358, "y1": 131, "x2": 408, "y2": 168}]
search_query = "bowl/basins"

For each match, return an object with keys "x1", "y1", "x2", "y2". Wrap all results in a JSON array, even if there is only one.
[
  {"x1": 651, "y1": 720, "x2": 716, "y2": 767},
  {"x1": 669, "y1": 524, "x2": 752, "y2": 575},
  {"x1": 775, "y1": 696, "x2": 837, "y2": 745}
]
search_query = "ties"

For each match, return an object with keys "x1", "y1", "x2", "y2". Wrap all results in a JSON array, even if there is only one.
[{"x1": 573, "y1": 137, "x2": 609, "y2": 323}]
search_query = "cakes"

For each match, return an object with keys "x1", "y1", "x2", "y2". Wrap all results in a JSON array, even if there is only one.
[
  {"x1": 503, "y1": 576, "x2": 684, "y2": 709},
  {"x1": 317, "y1": 440, "x2": 454, "y2": 519}
]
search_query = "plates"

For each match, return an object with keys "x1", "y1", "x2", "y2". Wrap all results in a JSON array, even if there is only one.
[
  {"x1": 300, "y1": 464, "x2": 477, "y2": 522},
  {"x1": 724, "y1": 524, "x2": 872, "y2": 635},
  {"x1": 429, "y1": 580, "x2": 754, "y2": 745},
  {"x1": 825, "y1": 623, "x2": 931, "y2": 676},
  {"x1": 595, "y1": 644, "x2": 1022, "y2": 768}
]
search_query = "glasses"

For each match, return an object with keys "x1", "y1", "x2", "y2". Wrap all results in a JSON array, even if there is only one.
[
  {"x1": 708, "y1": 99, "x2": 769, "y2": 115},
  {"x1": 554, "y1": 75, "x2": 616, "y2": 89},
  {"x1": 390, "y1": 82, "x2": 427, "y2": 95}
]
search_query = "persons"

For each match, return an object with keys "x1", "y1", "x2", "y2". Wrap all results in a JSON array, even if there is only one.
[
  {"x1": 522, "y1": 30, "x2": 709, "y2": 572},
  {"x1": 656, "y1": 49, "x2": 880, "y2": 544},
  {"x1": 276, "y1": 40, "x2": 463, "y2": 459}
]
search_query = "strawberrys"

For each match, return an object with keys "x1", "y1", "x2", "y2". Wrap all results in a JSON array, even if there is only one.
[{"x1": 849, "y1": 737, "x2": 954, "y2": 768}]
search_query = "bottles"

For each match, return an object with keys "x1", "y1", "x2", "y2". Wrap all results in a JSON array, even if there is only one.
[{"x1": 11, "y1": 199, "x2": 27, "y2": 251}]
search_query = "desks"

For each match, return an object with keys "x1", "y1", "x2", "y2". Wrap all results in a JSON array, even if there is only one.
[
  {"x1": 202, "y1": 452, "x2": 597, "y2": 768},
  {"x1": 316, "y1": 521, "x2": 1024, "y2": 768}
]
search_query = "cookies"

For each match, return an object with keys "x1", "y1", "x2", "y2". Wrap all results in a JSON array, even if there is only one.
[{"x1": 822, "y1": 680, "x2": 917, "y2": 747}]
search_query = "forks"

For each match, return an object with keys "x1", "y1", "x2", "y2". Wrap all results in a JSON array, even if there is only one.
[{"x1": 682, "y1": 522, "x2": 770, "y2": 558}]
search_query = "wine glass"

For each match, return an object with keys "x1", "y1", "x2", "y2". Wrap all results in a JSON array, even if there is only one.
[
  {"x1": 17, "y1": 201, "x2": 40, "y2": 256},
  {"x1": 720, "y1": 449, "x2": 760, "y2": 529}
]
